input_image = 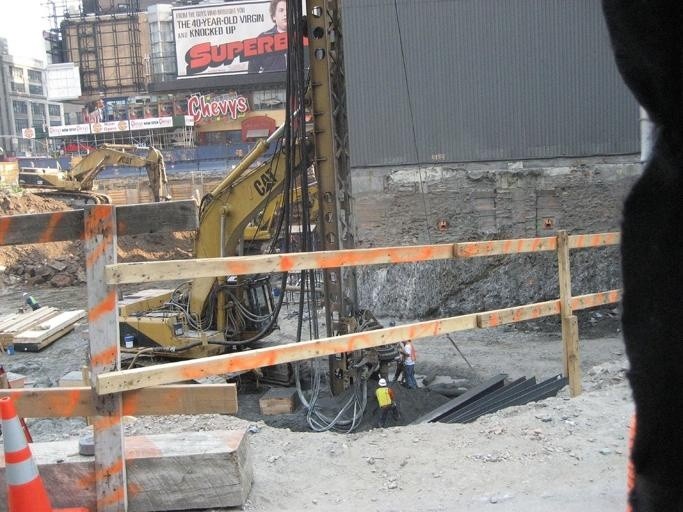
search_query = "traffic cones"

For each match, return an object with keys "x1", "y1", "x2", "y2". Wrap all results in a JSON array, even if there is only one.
[{"x1": 1, "y1": 394, "x2": 88, "y2": 511}]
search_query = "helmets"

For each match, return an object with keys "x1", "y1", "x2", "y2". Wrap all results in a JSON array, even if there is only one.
[{"x1": 379, "y1": 379, "x2": 387, "y2": 387}]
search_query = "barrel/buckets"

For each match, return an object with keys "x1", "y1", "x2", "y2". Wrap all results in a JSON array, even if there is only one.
[
  {"x1": 7, "y1": 341, "x2": 14, "y2": 354},
  {"x1": 124, "y1": 334, "x2": 133, "y2": 347}
]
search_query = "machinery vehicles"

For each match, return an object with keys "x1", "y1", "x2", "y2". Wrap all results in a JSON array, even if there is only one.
[
  {"x1": 18, "y1": 142, "x2": 172, "y2": 207},
  {"x1": 113, "y1": 0, "x2": 406, "y2": 397},
  {"x1": 243, "y1": 181, "x2": 321, "y2": 255}
]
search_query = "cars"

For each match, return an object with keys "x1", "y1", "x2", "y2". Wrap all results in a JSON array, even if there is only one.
[{"x1": 57, "y1": 140, "x2": 96, "y2": 155}]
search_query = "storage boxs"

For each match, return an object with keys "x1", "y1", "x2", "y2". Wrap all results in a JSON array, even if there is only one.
[{"x1": 259, "y1": 388, "x2": 301, "y2": 416}]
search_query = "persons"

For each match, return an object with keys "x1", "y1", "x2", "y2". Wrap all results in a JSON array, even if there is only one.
[
  {"x1": 375, "y1": 379, "x2": 400, "y2": 427},
  {"x1": 400, "y1": 340, "x2": 420, "y2": 389}
]
